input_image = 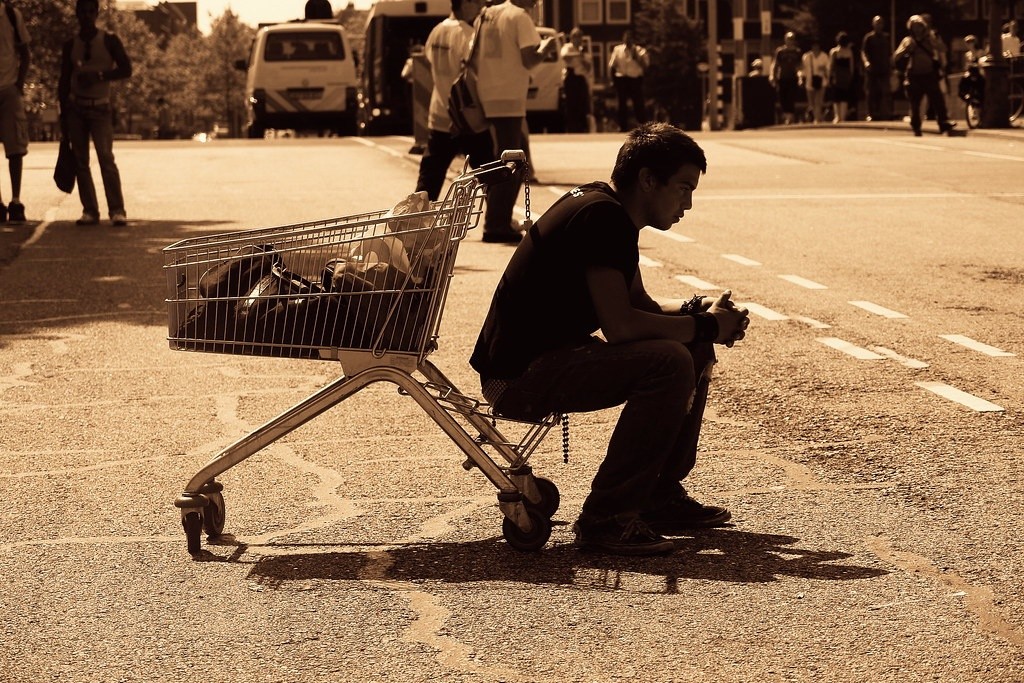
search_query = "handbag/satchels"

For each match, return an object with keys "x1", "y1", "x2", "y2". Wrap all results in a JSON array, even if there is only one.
[
  {"x1": 54, "y1": 113, "x2": 77, "y2": 193},
  {"x1": 174, "y1": 188, "x2": 448, "y2": 358},
  {"x1": 448, "y1": 71, "x2": 491, "y2": 133},
  {"x1": 812, "y1": 75, "x2": 823, "y2": 90}
]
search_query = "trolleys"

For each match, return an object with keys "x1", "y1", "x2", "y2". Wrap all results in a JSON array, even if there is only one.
[{"x1": 163, "y1": 147, "x2": 561, "y2": 553}]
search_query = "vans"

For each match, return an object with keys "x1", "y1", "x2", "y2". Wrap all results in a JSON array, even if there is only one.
[
  {"x1": 235, "y1": 25, "x2": 360, "y2": 138},
  {"x1": 526, "y1": 26, "x2": 562, "y2": 133}
]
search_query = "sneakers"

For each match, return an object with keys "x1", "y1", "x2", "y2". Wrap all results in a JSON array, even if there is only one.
[
  {"x1": 639, "y1": 493, "x2": 731, "y2": 526},
  {"x1": 573, "y1": 507, "x2": 674, "y2": 555}
]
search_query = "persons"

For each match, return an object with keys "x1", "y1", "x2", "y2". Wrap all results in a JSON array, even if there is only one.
[
  {"x1": 0, "y1": 0, "x2": 33, "y2": 225},
  {"x1": 416, "y1": 0, "x2": 1024, "y2": 246},
  {"x1": 468, "y1": 121, "x2": 750, "y2": 553},
  {"x1": 55, "y1": 0, "x2": 132, "y2": 225}
]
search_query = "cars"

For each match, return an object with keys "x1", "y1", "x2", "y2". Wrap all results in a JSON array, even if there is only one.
[{"x1": 958, "y1": 23, "x2": 1024, "y2": 129}]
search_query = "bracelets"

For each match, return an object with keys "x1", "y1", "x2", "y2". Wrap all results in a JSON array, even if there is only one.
[
  {"x1": 689, "y1": 312, "x2": 719, "y2": 344},
  {"x1": 680, "y1": 293, "x2": 706, "y2": 315},
  {"x1": 99, "y1": 72, "x2": 103, "y2": 82}
]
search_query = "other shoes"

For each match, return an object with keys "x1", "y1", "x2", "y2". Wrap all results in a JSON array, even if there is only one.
[
  {"x1": 8, "y1": 202, "x2": 27, "y2": 225},
  {"x1": 76, "y1": 212, "x2": 100, "y2": 225},
  {"x1": 482, "y1": 228, "x2": 523, "y2": 243},
  {"x1": 940, "y1": 123, "x2": 957, "y2": 133},
  {"x1": 912, "y1": 125, "x2": 922, "y2": 136},
  {"x1": 0, "y1": 203, "x2": 8, "y2": 224},
  {"x1": 111, "y1": 214, "x2": 127, "y2": 226}
]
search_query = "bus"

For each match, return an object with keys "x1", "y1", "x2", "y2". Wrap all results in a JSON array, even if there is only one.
[{"x1": 361, "y1": 1, "x2": 452, "y2": 135}]
